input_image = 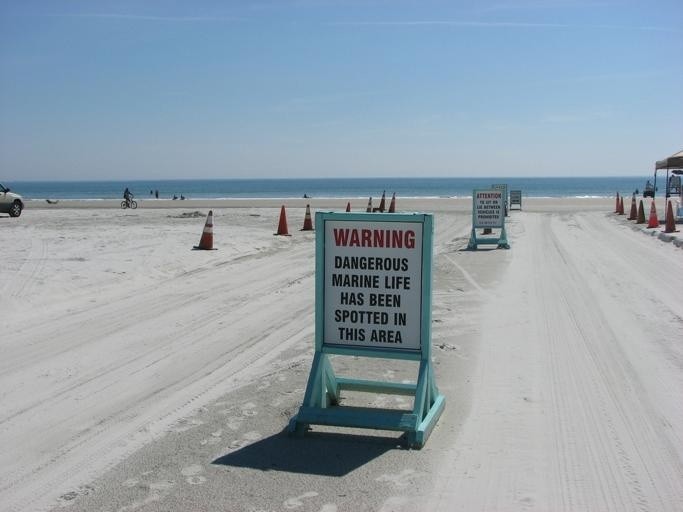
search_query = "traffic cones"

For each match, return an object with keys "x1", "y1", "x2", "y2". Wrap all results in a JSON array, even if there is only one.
[
  {"x1": 193, "y1": 210, "x2": 217, "y2": 250},
  {"x1": 346, "y1": 201, "x2": 350, "y2": 213},
  {"x1": 614, "y1": 192, "x2": 680, "y2": 234},
  {"x1": 300, "y1": 205, "x2": 315, "y2": 231},
  {"x1": 273, "y1": 205, "x2": 291, "y2": 237},
  {"x1": 366, "y1": 190, "x2": 396, "y2": 213}
]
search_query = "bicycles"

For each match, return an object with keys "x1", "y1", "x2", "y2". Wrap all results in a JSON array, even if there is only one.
[{"x1": 120, "y1": 196, "x2": 137, "y2": 209}]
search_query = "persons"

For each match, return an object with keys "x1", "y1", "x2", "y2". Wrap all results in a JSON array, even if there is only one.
[
  {"x1": 124, "y1": 188, "x2": 133, "y2": 208},
  {"x1": 645, "y1": 179, "x2": 653, "y2": 191}
]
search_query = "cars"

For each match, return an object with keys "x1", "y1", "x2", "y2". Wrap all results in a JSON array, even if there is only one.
[{"x1": 0, "y1": 183, "x2": 25, "y2": 217}]
